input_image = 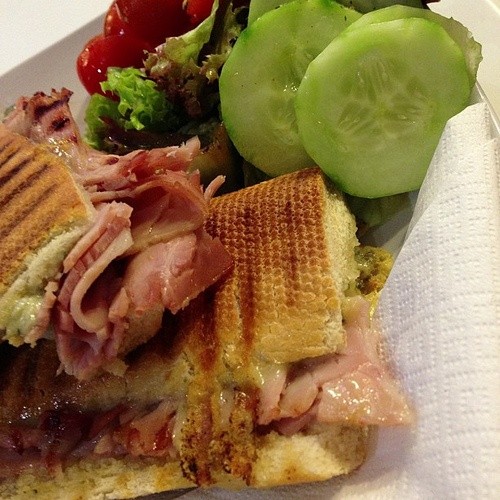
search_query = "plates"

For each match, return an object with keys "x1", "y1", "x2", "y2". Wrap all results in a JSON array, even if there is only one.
[{"x1": 0, "y1": 0, "x2": 498, "y2": 265}]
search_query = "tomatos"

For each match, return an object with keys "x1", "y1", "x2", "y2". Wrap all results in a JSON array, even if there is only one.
[{"x1": 76, "y1": 0, "x2": 213, "y2": 106}]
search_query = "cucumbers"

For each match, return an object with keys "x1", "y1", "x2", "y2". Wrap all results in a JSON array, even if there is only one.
[{"x1": 220, "y1": 0, "x2": 482, "y2": 198}]
